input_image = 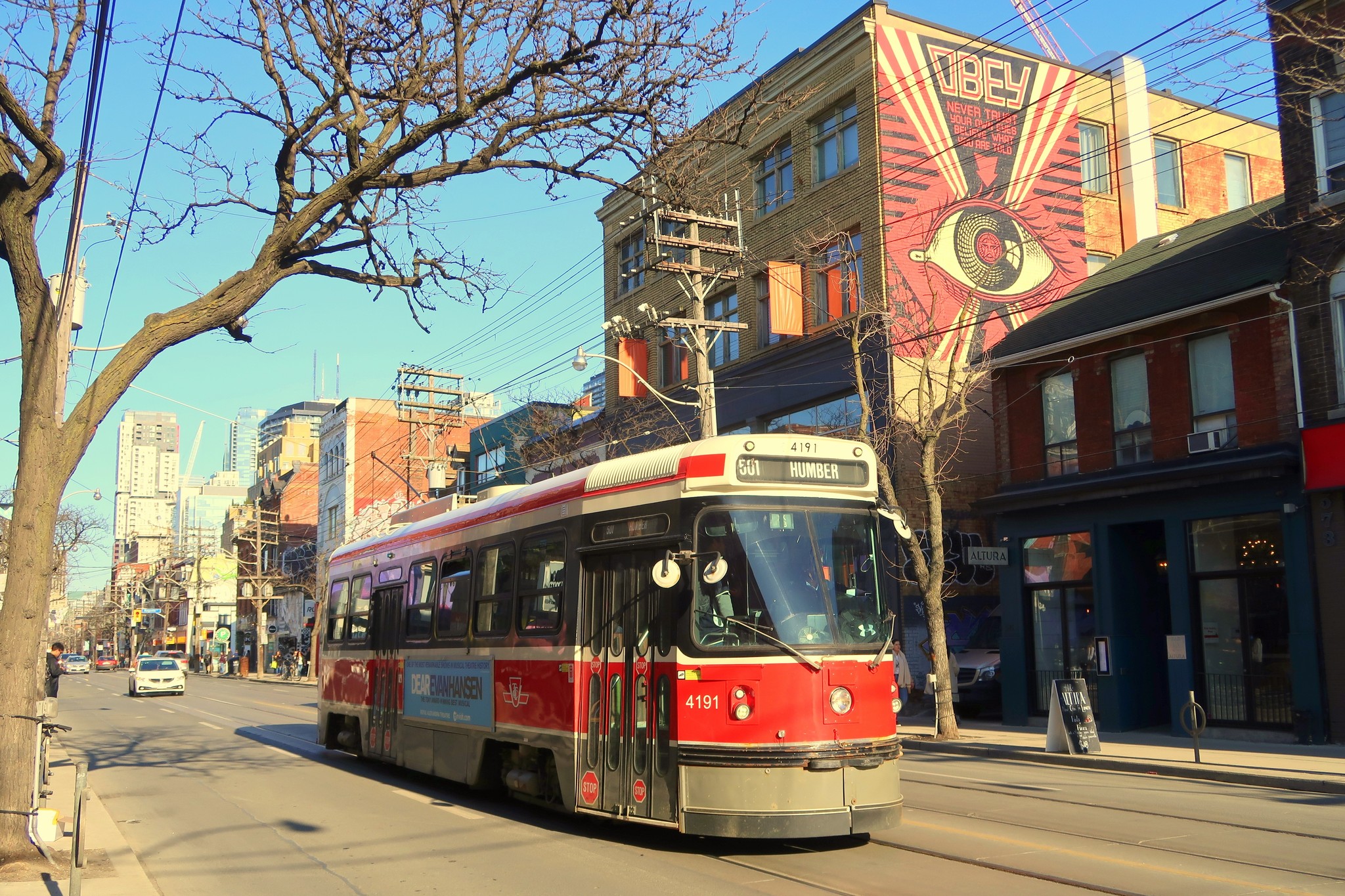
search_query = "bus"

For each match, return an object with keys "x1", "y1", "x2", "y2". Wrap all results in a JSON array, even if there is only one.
[{"x1": 306, "y1": 432, "x2": 912, "y2": 839}]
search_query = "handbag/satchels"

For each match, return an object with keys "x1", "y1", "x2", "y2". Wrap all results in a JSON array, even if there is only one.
[
  {"x1": 271, "y1": 660, "x2": 277, "y2": 669},
  {"x1": 923, "y1": 680, "x2": 934, "y2": 695}
]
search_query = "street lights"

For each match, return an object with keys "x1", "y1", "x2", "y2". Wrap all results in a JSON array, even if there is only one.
[{"x1": 573, "y1": 346, "x2": 720, "y2": 439}]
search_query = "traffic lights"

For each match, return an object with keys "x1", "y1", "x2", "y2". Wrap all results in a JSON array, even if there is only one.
[{"x1": 133, "y1": 609, "x2": 141, "y2": 623}]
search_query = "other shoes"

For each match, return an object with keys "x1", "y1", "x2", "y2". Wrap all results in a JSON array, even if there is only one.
[
  {"x1": 896, "y1": 721, "x2": 902, "y2": 727},
  {"x1": 932, "y1": 718, "x2": 935, "y2": 722}
]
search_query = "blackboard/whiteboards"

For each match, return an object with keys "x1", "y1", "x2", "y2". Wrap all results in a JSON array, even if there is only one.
[
  {"x1": 240, "y1": 656, "x2": 249, "y2": 674},
  {"x1": 1046, "y1": 678, "x2": 1103, "y2": 754}
]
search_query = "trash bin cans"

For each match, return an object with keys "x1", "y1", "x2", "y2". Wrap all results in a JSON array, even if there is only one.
[{"x1": 228, "y1": 656, "x2": 239, "y2": 674}]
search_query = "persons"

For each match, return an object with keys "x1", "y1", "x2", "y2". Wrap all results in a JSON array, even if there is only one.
[
  {"x1": 696, "y1": 532, "x2": 734, "y2": 630},
  {"x1": 118, "y1": 654, "x2": 130, "y2": 669},
  {"x1": 892, "y1": 639, "x2": 914, "y2": 727},
  {"x1": 923, "y1": 644, "x2": 959, "y2": 723},
  {"x1": 274, "y1": 647, "x2": 311, "y2": 678},
  {"x1": 793, "y1": 562, "x2": 865, "y2": 614},
  {"x1": 88, "y1": 658, "x2": 92, "y2": 664},
  {"x1": 204, "y1": 647, "x2": 250, "y2": 674},
  {"x1": 46, "y1": 643, "x2": 66, "y2": 697}
]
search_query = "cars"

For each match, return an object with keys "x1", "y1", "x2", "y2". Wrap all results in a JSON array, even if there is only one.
[
  {"x1": 57, "y1": 653, "x2": 78, "y2": 667},
  {"x1": 63, "y1": 655, "x2": 92, "y2": 675},
  {"x1": 128, "y1": 656, "x2": 186, "y2": 698},
  {"x1": 132, "y1": 649, "x2": 188, "y2": 680},
  {"x1": 95, "y1": 656, "x2": 117, "y2": 672}
]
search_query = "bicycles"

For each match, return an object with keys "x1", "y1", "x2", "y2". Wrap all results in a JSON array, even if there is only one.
[{"x1": 280, "y1": 662, "x2": 302, "y2": 681}]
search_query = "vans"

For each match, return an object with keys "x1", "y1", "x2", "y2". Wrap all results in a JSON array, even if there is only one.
[{"x1": 956, "y1": 600, "x2": 1033, "y2": 710}]
